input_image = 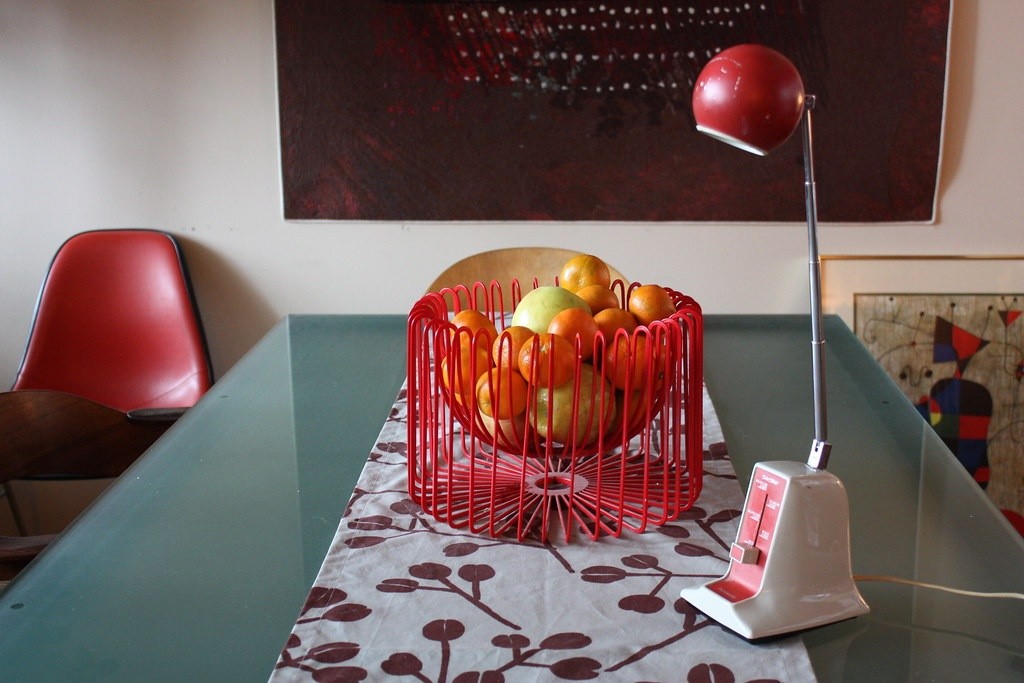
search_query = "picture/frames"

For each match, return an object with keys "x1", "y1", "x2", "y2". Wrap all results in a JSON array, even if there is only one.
[{"x1": 819, "y1": 254, "x2": 1024, "y2": 538}]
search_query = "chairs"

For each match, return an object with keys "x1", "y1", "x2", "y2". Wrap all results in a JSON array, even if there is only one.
[{"x1": 0, "y1": 227, "x2": 214, "y2": 581}]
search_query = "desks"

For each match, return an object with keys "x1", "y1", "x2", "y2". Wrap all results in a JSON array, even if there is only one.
[{"x1": 0, "y1": 316, "x2": 1024, "y2": 683}]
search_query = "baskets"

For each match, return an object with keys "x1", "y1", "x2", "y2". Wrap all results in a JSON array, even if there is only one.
[{"x1": 407, "y1": 277, "x2": 705, "y2": 546}]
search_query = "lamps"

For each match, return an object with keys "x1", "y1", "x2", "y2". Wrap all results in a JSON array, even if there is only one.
[{"x1": 680, "y1": 43, "x2": 870, "y2": 641}]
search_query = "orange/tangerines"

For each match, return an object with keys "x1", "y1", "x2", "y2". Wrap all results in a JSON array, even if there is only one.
[{"x1": 439, "y1": 254, "x2": 678, "y2": 419}]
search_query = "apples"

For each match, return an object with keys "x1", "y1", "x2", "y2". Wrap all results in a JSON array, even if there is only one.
[{"x1": 464, "y1": 285, "x2": 652, "y2": 449}]
